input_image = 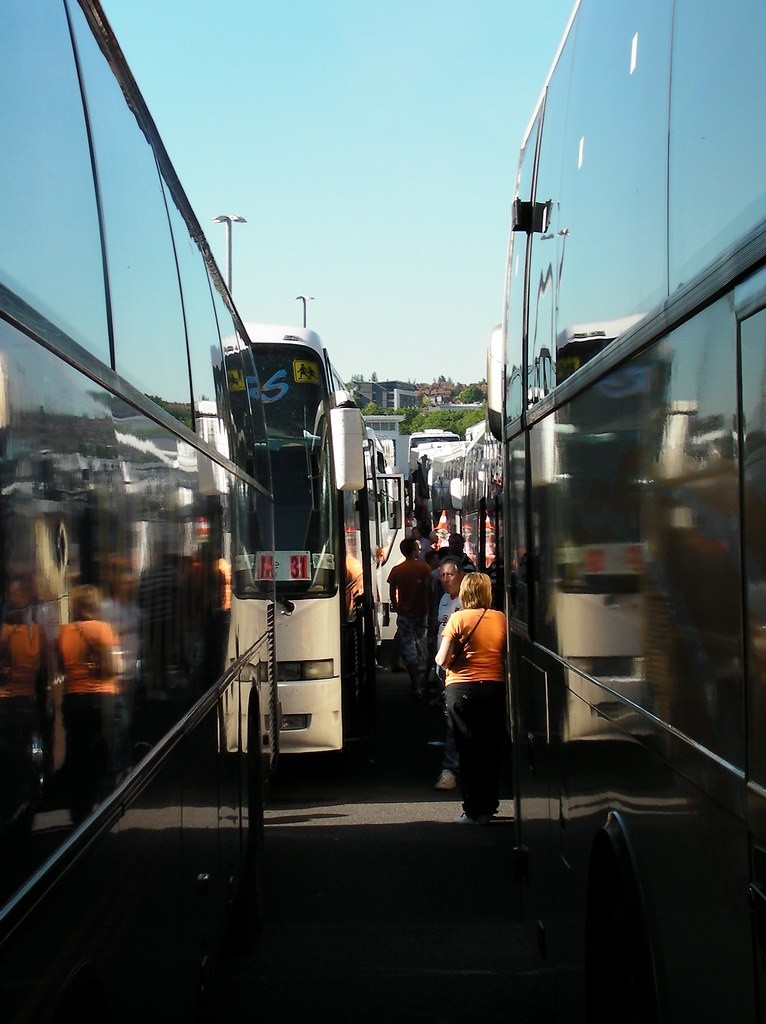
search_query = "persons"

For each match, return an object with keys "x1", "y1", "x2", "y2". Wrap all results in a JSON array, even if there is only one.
[
  {"x1": 0, "y1": 540, "x2": 232, "y2": 835},
  {"x1": 387, "y1": 510, "x2": 506, "y2": 826}
]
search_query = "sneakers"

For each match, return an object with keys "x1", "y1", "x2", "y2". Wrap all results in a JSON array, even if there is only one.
[{"x1": 434, "y1": 769, "x2": 457, "y2": 790}]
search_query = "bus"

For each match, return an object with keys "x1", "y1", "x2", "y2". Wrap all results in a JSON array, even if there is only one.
[
  {"x1": 484, "y1": 0, "x2": 765, "y2": 1024},
  {"x1": 234, "y1": 323, "x2": 508, "y2": 787},
  {"x1": 0, "y1": 0, "x2": 292, "y2": 1024}
]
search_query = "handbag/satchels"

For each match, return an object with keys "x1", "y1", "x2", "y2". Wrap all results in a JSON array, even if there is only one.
[
  {"x1": 436, "y1": 634, "x2": 464, "y2": 672},
  {"x1": 87, "y1": 646, "x2": 114, "y2": 680}
]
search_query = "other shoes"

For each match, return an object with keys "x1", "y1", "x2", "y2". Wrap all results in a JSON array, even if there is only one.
[
  {"x1": 412, "y1": 688, "x2": 421, "y2": 695},
  {"x1": 453, "y1": 812, "x2": 488, "y2": 825}
]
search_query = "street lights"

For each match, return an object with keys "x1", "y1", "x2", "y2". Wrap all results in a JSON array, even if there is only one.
[
  {"x1": 296, "y1": 294, "x2": 314, "y2": 329},
  {"x1": 213, "y1": 214, "x2": 247, "y2": 295}
]
